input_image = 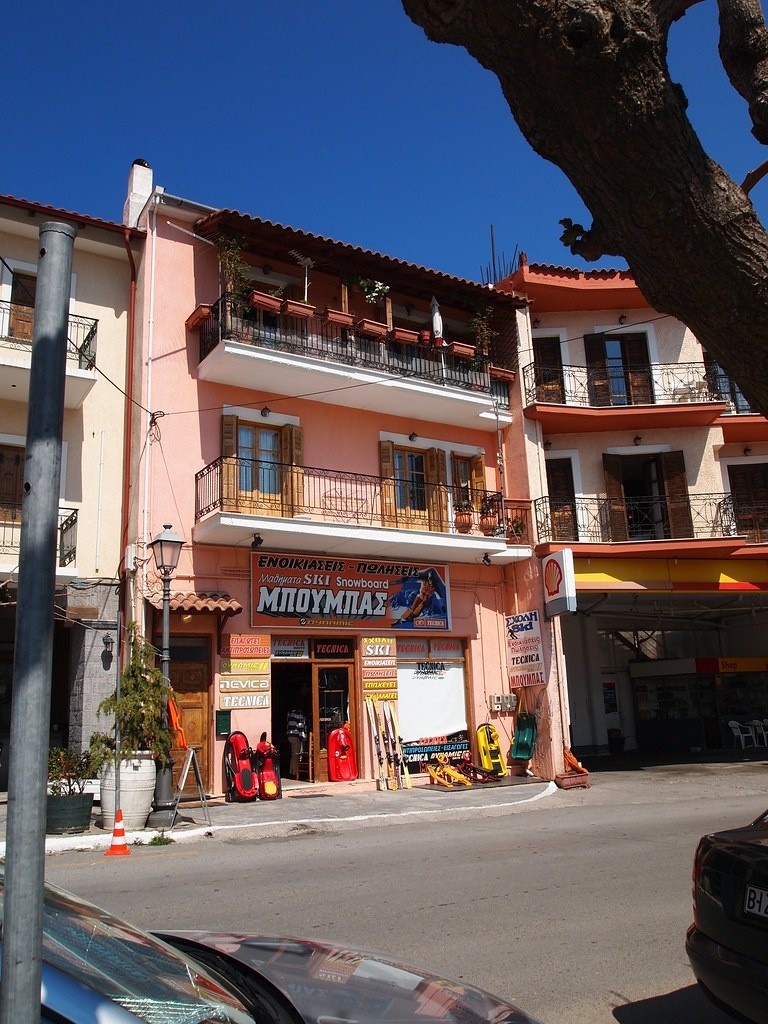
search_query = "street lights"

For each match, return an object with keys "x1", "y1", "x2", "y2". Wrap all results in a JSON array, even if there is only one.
[{"x1": 145, "y1": 522, "x2": 182, "y2": 829}]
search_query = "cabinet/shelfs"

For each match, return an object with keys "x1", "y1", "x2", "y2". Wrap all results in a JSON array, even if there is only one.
[{"x1": 320, "y1": 690, "x2": 344, "y2": 752}]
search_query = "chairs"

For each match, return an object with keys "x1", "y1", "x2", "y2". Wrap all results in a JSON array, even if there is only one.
[
  {"x1": 728, "y1": 719, "x2": 768, "y2": 752},
  {"x1": 295, "y1": 731, "x2": 314, "y2": 783}
]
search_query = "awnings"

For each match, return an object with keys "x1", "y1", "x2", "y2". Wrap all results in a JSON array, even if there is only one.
[{"x1": 146, "y1": 590, "x2": 243, "y2": 655}]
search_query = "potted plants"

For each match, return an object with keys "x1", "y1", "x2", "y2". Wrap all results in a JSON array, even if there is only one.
[
  {"x1": 280, "y1": 299, "x2": 317, "y2": 320},
  {"x1": 82, "y1": 620, "x2": 182, "y2": 833},
  {"x1": 46, "y1": 747, "x2": 97, "y2": 836},
  {"x1": 247, "y1": 290, "x2": 283, "y2": 315}
]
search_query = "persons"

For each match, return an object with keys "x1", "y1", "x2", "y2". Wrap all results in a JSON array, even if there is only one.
[{"x1": 287, "y1": 696, "x2": 307, "y2": 780}]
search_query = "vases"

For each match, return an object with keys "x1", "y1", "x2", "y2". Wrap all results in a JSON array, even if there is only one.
[
  {"x1": 322, "y1": 309, "x2": 356, "y2": 329},
  {"x1": 356, "y1": 318, "x2": 389, "y2": 340},
  {"x1": 508, "y1": 533, "x2": 523, "y2": 546},
  {"x1": 447, "y1": 341, "x2": 477, "y2": 360},
  {"x1": 364, "y1": 275, "x2": 378, "y2": 288},
  {"x1": 454, "y1": 511, "x2": 473, "y2": 533},
  {"x1": 478, "y1": 515, "x2": 499, "y2": 537},
  {"x1": 488, "y1": 366, "x2": 516, "y2": 384},
  {"x1": 389, "y1": 327, "x2": 421, "y2": 346},
  {"x1": 185, "y1": 303, "x2": 214, "y2": 331},
  {"x1": 555, "y1": 770, "x2": 588, "y2": 790}
]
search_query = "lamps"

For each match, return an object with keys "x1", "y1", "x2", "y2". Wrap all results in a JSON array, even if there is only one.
[
  {"x1": 250, "y1": 534, "x2": 264, "y2": 551},
  {"x1": 409, "y1": 431, "x2": 419, "y2": 442},
  {"x1": 619, "y1": 314, "x2": 627, "y2": 325},
  {"x1": 481, "y1": 553, "x2": 491, "y2": 567},
  {"x1": 544, "y1": 440, "x2": 553, "y2": 451},
  {"x1": 533, "y1": 318, "x2": 541, "y2": 329},
  {"x1": 101, "y1": 630, "x2": 115, "y2": 652},
  {"x1": 744, "y1": 446, "x2": 751, "y2": 457},
  {"x1": 260, "y1": 405, "x2": 272, "y2": 419},
  {"x1": 633, "y1": 434, "x2": 642, "y2": 446}
]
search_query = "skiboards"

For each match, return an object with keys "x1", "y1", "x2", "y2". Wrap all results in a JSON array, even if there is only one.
[
  {"x1": 365, "y1": 693, "x2": 397, "y2": 791},
  {"x1": 383, "y1": 701, "x2": 411, "y2": 790}
]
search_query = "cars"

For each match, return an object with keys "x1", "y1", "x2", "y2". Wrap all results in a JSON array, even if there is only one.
[
  {"x1": 684, "y1": 808, "x2": 768, "y2": 1024},
  {"x1": 0, "y1": 850, "x2": 546, "y2": 1024}
]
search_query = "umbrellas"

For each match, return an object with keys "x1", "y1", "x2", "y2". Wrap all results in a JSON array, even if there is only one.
[{"x1": 430, "y1": 295, "x2": 443, "y2": 337}]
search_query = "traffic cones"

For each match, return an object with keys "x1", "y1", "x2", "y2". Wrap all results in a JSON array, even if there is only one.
[{"x1": 104, "y1": 808, "x2": 133, "y2": 855}]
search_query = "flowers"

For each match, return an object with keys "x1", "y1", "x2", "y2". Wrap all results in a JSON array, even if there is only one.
[
  {"x1": 358, "y1": 278, "x2": 390, "y2": 305},
  {"x1": 479, "y1": 494, "x2": 502, "y2": 516},
  {"x1": 452, "y1": 498, "x2": 475, "y2": 513},
  {"x1": 506, "y1": 516, "x2": 526, "y2": 535}
]
